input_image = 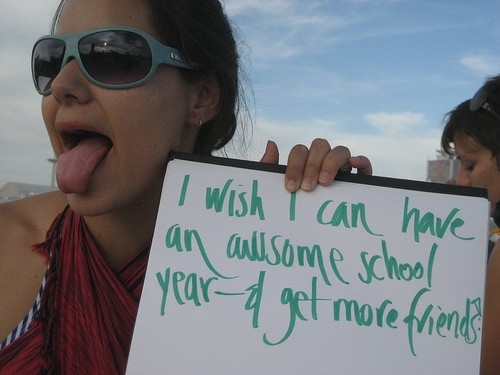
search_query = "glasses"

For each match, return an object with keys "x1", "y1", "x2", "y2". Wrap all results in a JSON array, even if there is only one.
[
  {"x1": 469, "y1": 80, "x2": 500, "y2": 121},
  {"x1": 30, "y1": 26, "x2": 200, "y2": 97}
]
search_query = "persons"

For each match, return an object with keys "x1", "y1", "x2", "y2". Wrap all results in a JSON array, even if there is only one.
[
  {"x1": 439, "y1": 74, "x2": 500, "y2": 374},
  {"x1": 0, "y1": 0, "x2": 373, "y2": 375}
]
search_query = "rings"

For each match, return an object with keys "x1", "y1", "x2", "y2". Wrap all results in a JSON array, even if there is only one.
[{"x1": 339, "y1": 166, "x2": 352, "y2": 174}]
